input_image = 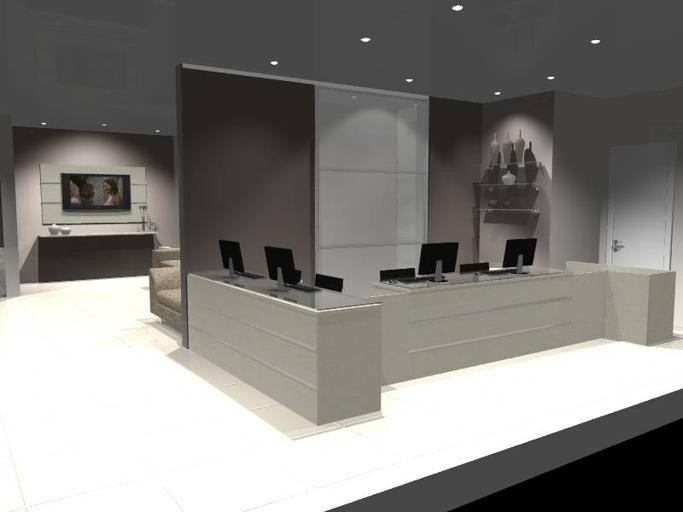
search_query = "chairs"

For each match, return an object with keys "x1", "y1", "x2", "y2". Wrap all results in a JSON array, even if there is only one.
[
  {"x1": 150, "y1": 246, "x2": 181, "y2": 328},
  {"x1": 313, "y1": 262, "x2": 491, "y2": 293}
]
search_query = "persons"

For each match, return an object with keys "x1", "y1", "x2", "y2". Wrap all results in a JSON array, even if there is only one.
[
  {"x1": 103, "y1": 179, "x2": 121, "y2": 206},
  {"x1": 70, "y1": 175, "x2": 87, "y2": 206}
]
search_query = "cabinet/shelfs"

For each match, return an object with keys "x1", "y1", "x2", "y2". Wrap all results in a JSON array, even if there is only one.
[{"x1": 473, "y1": 160, "x2": 542, "y2": 214}]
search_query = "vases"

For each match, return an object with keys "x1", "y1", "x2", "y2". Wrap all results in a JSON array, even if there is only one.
[
  {"x1": 48, "y1": 221, "x2": 72, "y2": 235},
  {"x1": 490, "y1": 128, "x2": 525, "y2": 166}
]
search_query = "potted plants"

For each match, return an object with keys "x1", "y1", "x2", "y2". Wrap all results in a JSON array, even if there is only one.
[{"x1": 139, "y1": 205, "x2": 147, "y2": 231}]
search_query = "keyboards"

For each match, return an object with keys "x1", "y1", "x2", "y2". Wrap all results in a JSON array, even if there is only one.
[
  {"x1": 285, "y1": 283, "x2": 322, "y2": 292},
  {"x1": 234, "y1": 270, "x2": 265, "y2": 279},
  {"x1": 397, "y1": 275, "x2": 445, "y2": 284},
  {"x1": 482, "y1": 268, "x2": 516, "y2": 275}
]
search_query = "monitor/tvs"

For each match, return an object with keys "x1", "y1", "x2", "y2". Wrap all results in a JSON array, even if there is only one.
[
  {"x1": 264, "y1": 245, "x2": 297, "y2": 292},
  {"x1": 417, "y1": 241, "x2": 459, "y2": 282},
  {"x1": 503, "y1": 237, "x2": 538, "y2": 275},
  {"x1": 218, "y1": 238, "x2": 245, "y2": 278}
]
